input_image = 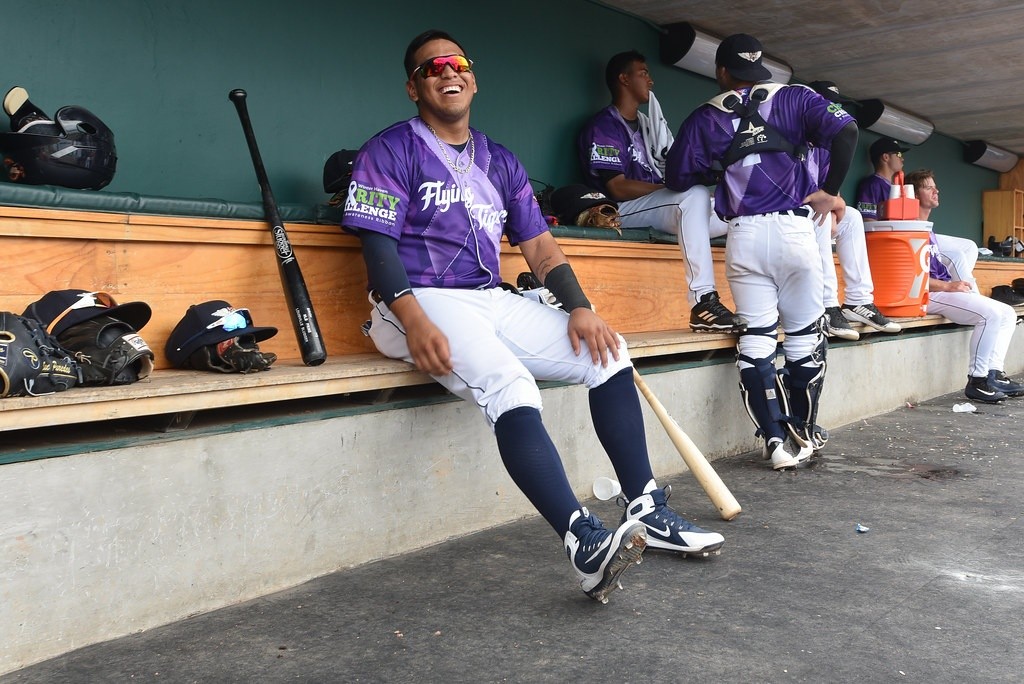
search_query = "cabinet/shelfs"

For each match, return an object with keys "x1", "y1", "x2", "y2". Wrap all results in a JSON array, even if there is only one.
[{"x1": 982, "y1": 188, "x2": 1024, "y2": 260}]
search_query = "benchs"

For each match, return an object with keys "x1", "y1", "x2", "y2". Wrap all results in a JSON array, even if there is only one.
[{"x1": 0, "y1": 185, "x2": 1024, "y2": 438}]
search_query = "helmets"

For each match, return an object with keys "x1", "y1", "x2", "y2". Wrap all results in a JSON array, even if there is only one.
[
  {"x1": 498, "y1": 271, "x2": 596, "y2": 317},
  {"x1": 987, "y1": 235, "x2": 1013, "y2": 257},
  {"x1": 0, "y1": 105, "x2": 118, "y2": 190}
]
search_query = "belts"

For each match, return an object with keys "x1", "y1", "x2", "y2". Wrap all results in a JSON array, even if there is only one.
[{"x1": 725, "y1": 207, "x2": 809, "y2": 218}]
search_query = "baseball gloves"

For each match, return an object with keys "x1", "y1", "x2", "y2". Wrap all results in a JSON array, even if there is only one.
[
  {"x1": 0, "y1": 311, "x2": 156, "y2": 397},
  {"x1": 572, "y1": 202, "x2": 625, "y2": 231},
  {"x1": 1, "y1": 86, "x2": 52, "y2": 134},
  {"x1": 499, "y1": 271, "x2": 597, "y2": 315},
  {"x1": 180, "y1": 332, "x2": 278, "y2": 375}
]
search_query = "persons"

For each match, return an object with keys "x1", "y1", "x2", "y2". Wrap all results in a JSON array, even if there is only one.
[
  {"x1": 856, "y1": 136, "x2": 981, "y2": 294},
  {"x1": 811, "y1": 206, "x2": 902, "y2": 340},
  {"x1": 577, "y1": 48, "x2": 750, "y2": 333},
  {"x1": 665, "y1": 32, "x2": 860, "y2": 472},
  {"x1": 340, "y1": 29, "x2": 725, "y2": 605},
  {"x1": 904, "y1": 168, "x2": 1024, "y2": 403}
]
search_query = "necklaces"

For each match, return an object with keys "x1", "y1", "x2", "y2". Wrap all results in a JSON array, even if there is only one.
[{"x1": 427, "y1": 125, "x2": 475, "y2": 174}]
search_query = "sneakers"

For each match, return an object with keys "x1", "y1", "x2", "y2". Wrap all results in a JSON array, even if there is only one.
[
  {"x1": 765, "y1": 435, "x2": 814, "y2": 473},
  {"x1": 964, "y1": 374, "x2": 1009, "y2": 404},
  {"x1": 563, "y1": 506, "x2": 649, "y2": 605},
  {"x1": 688, "y1": 292, "x2": 749, "y2": 335},
  {"x1": 989, "y1": 277, "x2": 1024, "y2": 307},
  {"x1": 987, "y1": 369, "x2": 1024, "y2": 398},
  {"x1": 615, "y1": 484, "x2": 726, "y2": 559},
  {"x1": 798, "y1": 431, "x2": 826, "y2": 451},
  {"x1": 840, "y1": 302, "x2": 901, "y2": 333},
  {"x1": 824, "y1": 306, "x2": 860, "y2": 341}
]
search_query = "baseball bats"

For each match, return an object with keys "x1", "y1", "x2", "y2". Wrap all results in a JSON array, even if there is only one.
[
  {"x1": 631, "y1": 367, "x2": 742, "y2": 521},
  {"x1": 228, "y1": 88, "x2": 329, "y2": 367}
]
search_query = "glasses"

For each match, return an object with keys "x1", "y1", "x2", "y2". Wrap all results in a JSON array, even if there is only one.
[
  {"x1": 177, "y1": 308, "x2": 253, "y2": 351},
  {"x1": 410, "y1": 54, "x2": 474, "y2": 81},
  {"x1": 46, "y1": 291, "x2": 122, "y2": 335},
  {"x1": 886, "y1": 151, "x2": 904, "y2": 158}
]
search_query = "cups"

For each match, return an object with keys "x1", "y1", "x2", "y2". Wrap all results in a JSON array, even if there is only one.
[{"x1": 592, "y1": 477, "x2": 621, "y2": 501}]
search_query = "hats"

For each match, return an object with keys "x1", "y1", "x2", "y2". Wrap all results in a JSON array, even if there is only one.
[
  {"x1": 322, "y1": 148, "x2": 359, "y2": 194},
  {"x1": 807, "y1": 79, "x2": 855, "y2": 105},
  {"x1": 869, "y1": 137, "x2": 911, "y2": 160},
  {"x1": 163, "y1": 299, "x2": 280, "y2": 369},
  {"x1": 550, "y1": 183, "x2": 620, "y2": 222},
  {"x1": 21, "y1": 289, "x2": 153, "y2": 337},
  {"x1": 715, "y1": 32, "x2": 773, "y2": 82}
]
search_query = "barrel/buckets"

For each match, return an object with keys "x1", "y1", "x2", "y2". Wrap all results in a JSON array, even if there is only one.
[{"x1": 863, "y1": 220, "x2": 934, "y2": 316}]
